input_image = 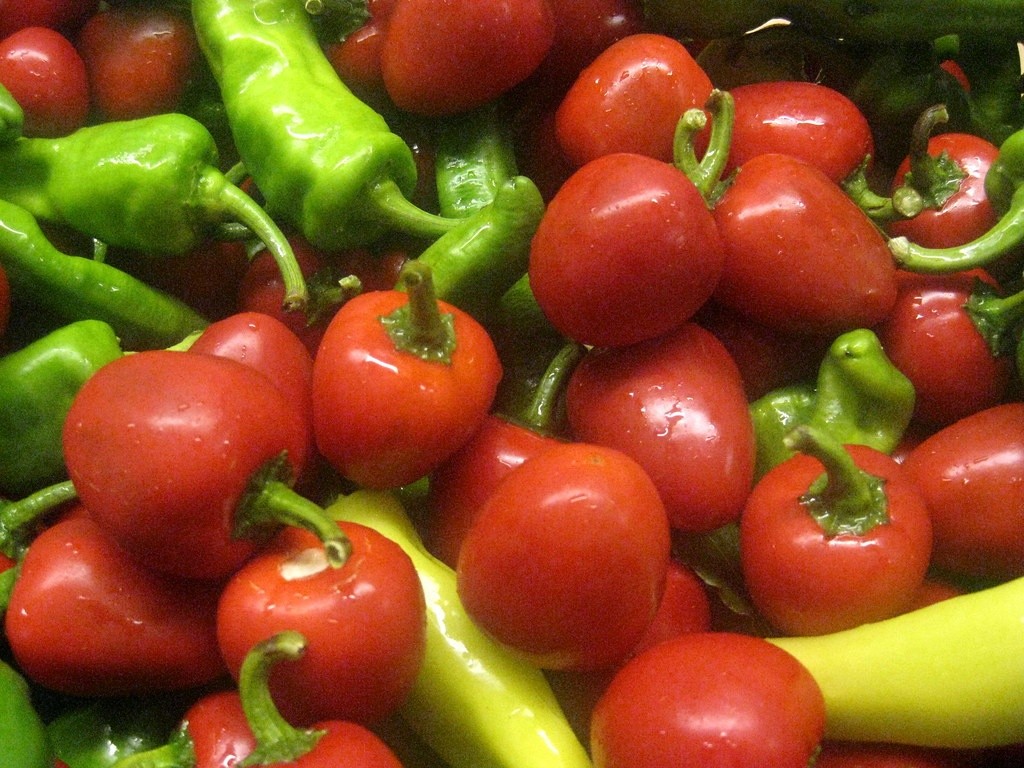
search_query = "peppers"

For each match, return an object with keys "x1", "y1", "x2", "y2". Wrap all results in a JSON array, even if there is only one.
[{"x1": 0, "y1": 0, "x2": 1024, "y2": 768}]
